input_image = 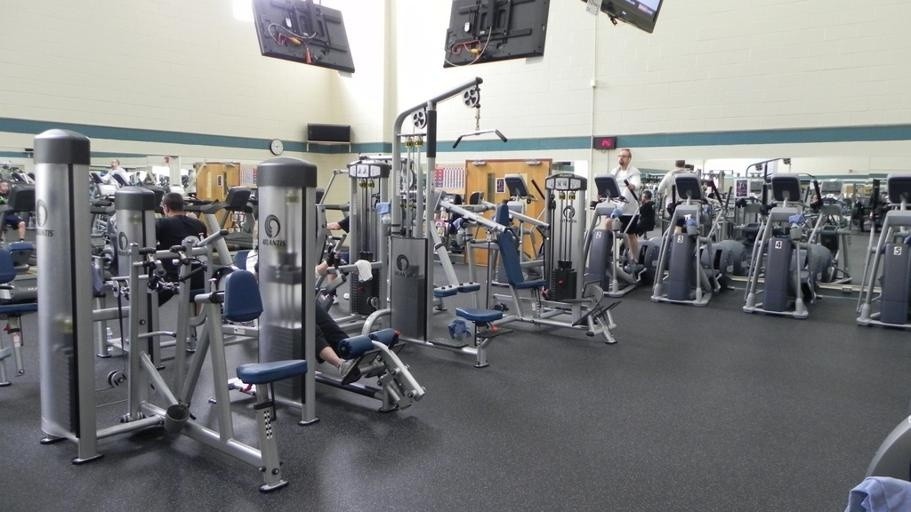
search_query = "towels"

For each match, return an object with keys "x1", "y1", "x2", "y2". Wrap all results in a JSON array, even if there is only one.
[{"x1": 355, "y1": 260, "x2": 373, "y2": 281}]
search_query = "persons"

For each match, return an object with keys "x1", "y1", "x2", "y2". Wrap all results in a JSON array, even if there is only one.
[
  {"x1": 326, "y1": 211, "x2": 352, "y2": 235},
  {"x1": 658, "y1": 160, "x2": 703, "y2": 233},
  {"x1": 603, "y1": 148, "x2": 643, "y2": 265},
  {"x1": 0, "y1": 177, "x2": 25, "y2": 244},
  {"x1": 446, "y1": 193, "x2": 464, "y2": 247},
  {"x1": 631, "y1": 190, "x2": 655, "y2": 232},
  {"x1": 150, "y1": 191, "x2": 211, "y2": 309},
  {"x1": 98, "y1": 159, "x2": 132, "y2": 190},
  {"x1": 304, "y1": 248, "x2": 371, "y2": 376}
]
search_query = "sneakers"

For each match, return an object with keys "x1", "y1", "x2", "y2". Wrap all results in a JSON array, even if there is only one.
[
  {"x1": 185, "y1": 335, "x2": 197, "y2": 356},
  {"x1": 337, "y1": 357, "x2": 364, "y2": 385}
]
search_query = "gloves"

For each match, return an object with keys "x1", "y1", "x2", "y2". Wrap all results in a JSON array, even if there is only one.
[{"x1": 324, "y1": 251, "x2": 340, "y2": 267}]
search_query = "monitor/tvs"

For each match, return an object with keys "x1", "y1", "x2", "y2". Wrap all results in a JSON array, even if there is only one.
[
  {"x1": 585, "y1": 1, "x2": 665, "y2": 34},
  {"x1": 442, "y1": 0, "x2": 549, "y2": 69},
  {"x1": 252, "y1": 1, "x2": 355, "y2": 73}
]
